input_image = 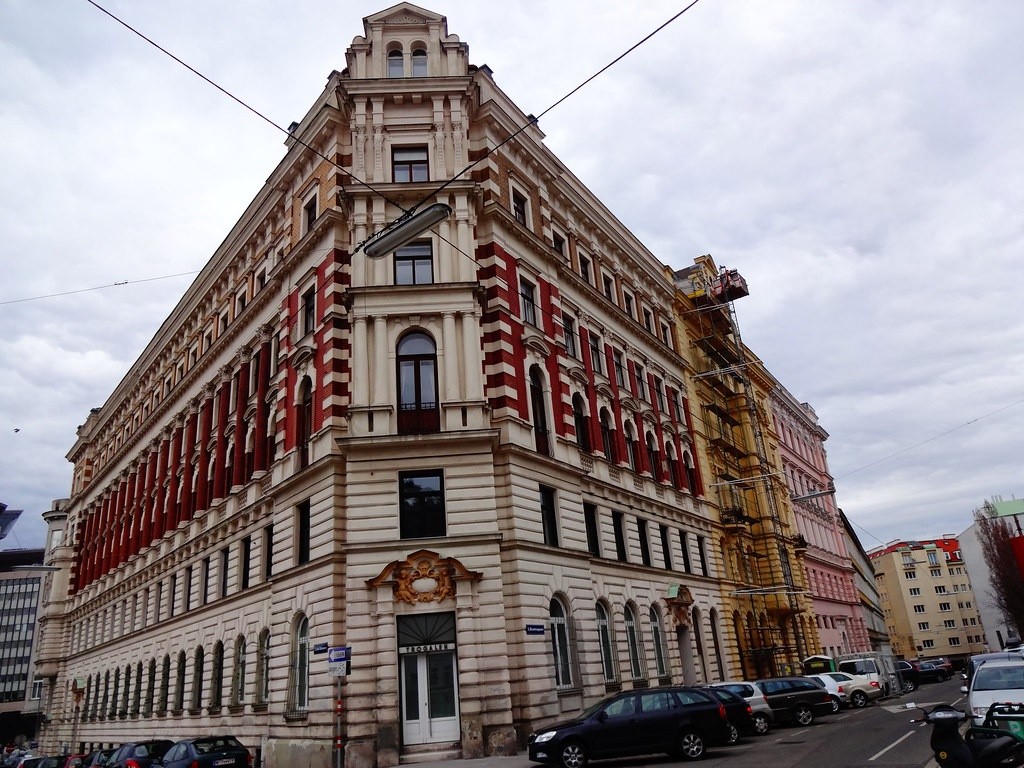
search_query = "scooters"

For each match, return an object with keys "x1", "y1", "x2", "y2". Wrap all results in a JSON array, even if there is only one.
[{"x1": 905, "y1": 702, "x2": 1024, "y2": 768}]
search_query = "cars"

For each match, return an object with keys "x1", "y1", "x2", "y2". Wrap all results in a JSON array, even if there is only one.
[
  {"x1": 101, "y1": 739, "x2": 181, "y2": 768},
  {"x1": 915, "y1": 659, "x2": 954, "y2": 683},
  {"x1": 959, "y1": 652, "x2": 1024, "y2": 685},
  {"x1": 1, "y1": 748, "x2": 137, "y2": 768},
  {"x1": 152, "y1": 734, "x2": 252, "y2": 768},
  {"x1": 751, "y1": 677, "x2": 834, "y2": 727},
  {"x1": 526, "y1": 685, "x2": 729, "y2": 768},
  {"x1": 823, "y1": 671, "x2": 882, "y2": 709},
  {"x1": 801, "y1": 674, "x2": 847, "y2": 715},
  {"x1": 893, "y1": 660, "x2": 922, "y2": 693},
  {"x1": 645, "y1": 687, "x2": 757, "y2": 746},
  {"x1": 959, "y1": 660, "x2": 1024, "y2": 729},
  {"x1": 838, "y1": 657, "x2": 887, "y2": 699},
  {"x1": 709, "y1": 682, "x2": 775, "y2": 737}
]
